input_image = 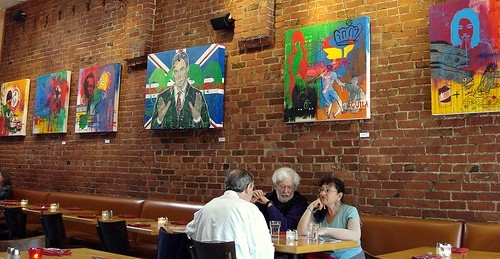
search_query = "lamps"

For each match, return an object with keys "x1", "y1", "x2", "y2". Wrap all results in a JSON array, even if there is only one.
[{"x1": 210, "y1": 12, "x2": 233, "y2": 31}]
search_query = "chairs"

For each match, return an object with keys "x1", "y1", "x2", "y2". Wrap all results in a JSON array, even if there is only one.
[
  {"x1": 157, "y1": 227, "x2": 236, "y2": 259},
  {"x1": 96, "y1": 220, "x2": 130, "y2": 257},
  {"x1": 40, "y1": 213, "x2": 67, "y2": 250}
]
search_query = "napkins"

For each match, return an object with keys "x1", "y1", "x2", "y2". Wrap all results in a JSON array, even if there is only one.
[
  {"x1": 128, "y1": 222, "x2": 152, "y2": 227},
  {"x1": 451, "y1": 247, "x2": 469, "y2": 254},
  {"x1": 412, "y1": 255, "x2": 443, "y2": 259},
  {"x1": 77, "y1": 214, "x2": 100, "y2": 219},
  {"x1": 170, "y1": 221, "x2": 189, "y2": 225},
  {"x1": 119, "y1": 214, "x2": 137, "y2": 219},
  {"x1": 42, "y1": 247, "x2": 71, "y2": 256}
]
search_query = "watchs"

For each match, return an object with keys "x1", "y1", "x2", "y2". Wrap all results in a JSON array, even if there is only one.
[{"x1": 266, "y1": 200, "x2": 273, "y2": 207}]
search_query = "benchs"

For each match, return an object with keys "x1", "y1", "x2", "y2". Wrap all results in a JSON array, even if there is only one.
[{"x1": 12, "y1": 189, "x2": 500, "y2": 259}]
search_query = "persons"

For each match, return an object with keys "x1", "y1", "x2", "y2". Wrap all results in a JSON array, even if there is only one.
[
  {"x1": 184, "y1": 168, "x2": 274, "y2": 259},
  {"x1": 297, "y1": 177, "x2": 365, "y2": 259},
  {"x1": 253, "y1": 167, "x2": 308, "y2": 232},
  {"x1": 0, "y1": 168, "x2": 14, "y2": 200}
]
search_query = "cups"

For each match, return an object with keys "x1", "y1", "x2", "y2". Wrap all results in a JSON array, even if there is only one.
[
  {"x1": 307, "y1": 222, "x2": 319, "y2": 241},
  {"x1": 269, "y1": 221, "x2": 281, "y2": 238},
  {"x1": 29, "y1": 247, "x2": 42, "y2": 259}
]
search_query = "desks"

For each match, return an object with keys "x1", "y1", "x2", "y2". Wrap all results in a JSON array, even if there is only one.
[
  {"x1": 60, "y1": 214, "x2": 154, "y2": 223},
  {"x1": 22, "y1": 207, "x2": 99, "y2": 213},
  {"x1": 0, "y1": 248, "x2": 142, "y2": 259},
  {"x1": 127, "y1": 221, "x2": 186, "y2": 235},
  {"x1": 375, "y1": 246, "x2": 500, "y2": 259},
  {"x1": 3, "y1": 207, "x2": 27, "y2": 242},
  {"x1": 273, "y1": 235, "x2": 358, "y2": 259}
]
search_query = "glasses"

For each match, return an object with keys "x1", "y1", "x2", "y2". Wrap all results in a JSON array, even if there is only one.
[{"x1": 319, "y1": 188, "x2": 338, "y2": 193}]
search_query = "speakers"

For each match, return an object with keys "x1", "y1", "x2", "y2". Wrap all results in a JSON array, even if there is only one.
[
  {"x1": 10, "y1": 10, "x2": 24, "y2": 21},
  {"x1": 209, "y1": 12, "x2": 233, "y2": 31}
]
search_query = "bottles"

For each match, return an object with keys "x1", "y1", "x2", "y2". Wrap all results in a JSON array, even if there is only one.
[
  {"x1": 288, "y1": 229, "x2": 298, "y2": 240},
  {"x1": 6, "y1": 246, "x2": 20, "y2": 259}
]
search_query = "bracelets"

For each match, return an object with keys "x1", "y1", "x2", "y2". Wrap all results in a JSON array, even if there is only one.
[{"x1": 307, "y1": 207, "x2": 313, "y2": 212}]
